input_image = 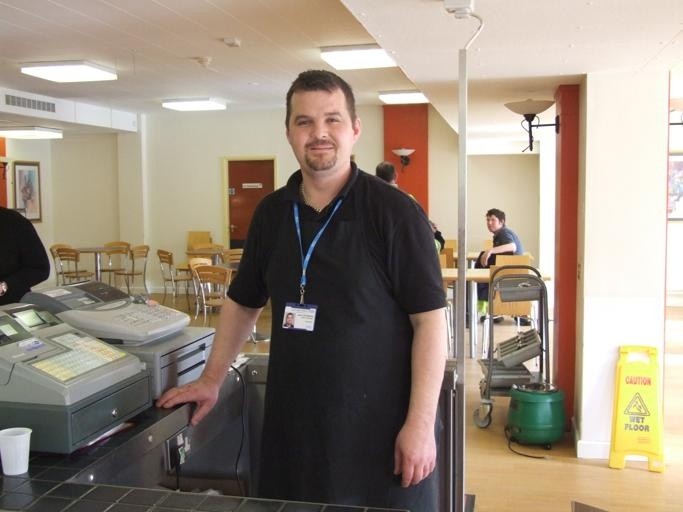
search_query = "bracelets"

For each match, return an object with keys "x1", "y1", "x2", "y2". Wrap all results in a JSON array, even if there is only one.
[{"x1": 0, "y1": 282, "x2": 7, "y2": 298}]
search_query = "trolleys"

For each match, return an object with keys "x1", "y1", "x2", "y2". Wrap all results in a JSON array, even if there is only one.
[{"x1": 472, "y1": 264, "x2": 550, "y2": 429}]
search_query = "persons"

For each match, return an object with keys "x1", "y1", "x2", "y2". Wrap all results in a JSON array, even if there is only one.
[
  {"x1": 374, "y1": 160, "x2": 405, "y2": 191},
  {"x1": 0, "y1": 206, "x2": 50, "y2": 305},
  {"x1": 479, "y1": 209, "x2": 532, "y2": 327},
  {"x1": 154, "y1": 70, "x2": 447, "y2": 512},
  {"x1": 431, "y1": 230, "x2": 445, "y2": 255}
]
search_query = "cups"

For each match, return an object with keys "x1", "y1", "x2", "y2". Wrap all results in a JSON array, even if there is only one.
[{"x1": 0, "y1": 426, "x2": 32, "y2": 476}]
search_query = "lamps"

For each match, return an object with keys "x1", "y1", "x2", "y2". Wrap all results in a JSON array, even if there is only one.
[
  {"x1": 19, "y1": 60, "x2": 119, "y2": 83},
  {"x1": 320, "y1": 43, "x2": 398, "y2": 70},
  {"x1": 377, "y1": 90, "x2": 430, "y2": 105},
  {"x1": 160, "y1": 98, "x2": 226, "y2": 112},
  {"x1": 391, "y1": 148, "x2": 416, "y2": 172},
  {"x1": 0, "y1": 126, "x2": 62, "y2": 142},
  {"x1": 503, "y1": 97, "x2": 559, "y2": 154}
]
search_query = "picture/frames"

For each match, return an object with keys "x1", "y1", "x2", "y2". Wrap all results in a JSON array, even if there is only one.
[{"x1": 11, "y1": 160, "x2": 43, "y2": 223}]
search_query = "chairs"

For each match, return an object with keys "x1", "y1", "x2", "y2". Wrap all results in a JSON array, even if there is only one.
[
  {"x1": 439, "y1": 239, "x2": 554, "y2": 361},
  {"x1": 50, "y1": 230, "x2": 273, "y2": 343}
]
